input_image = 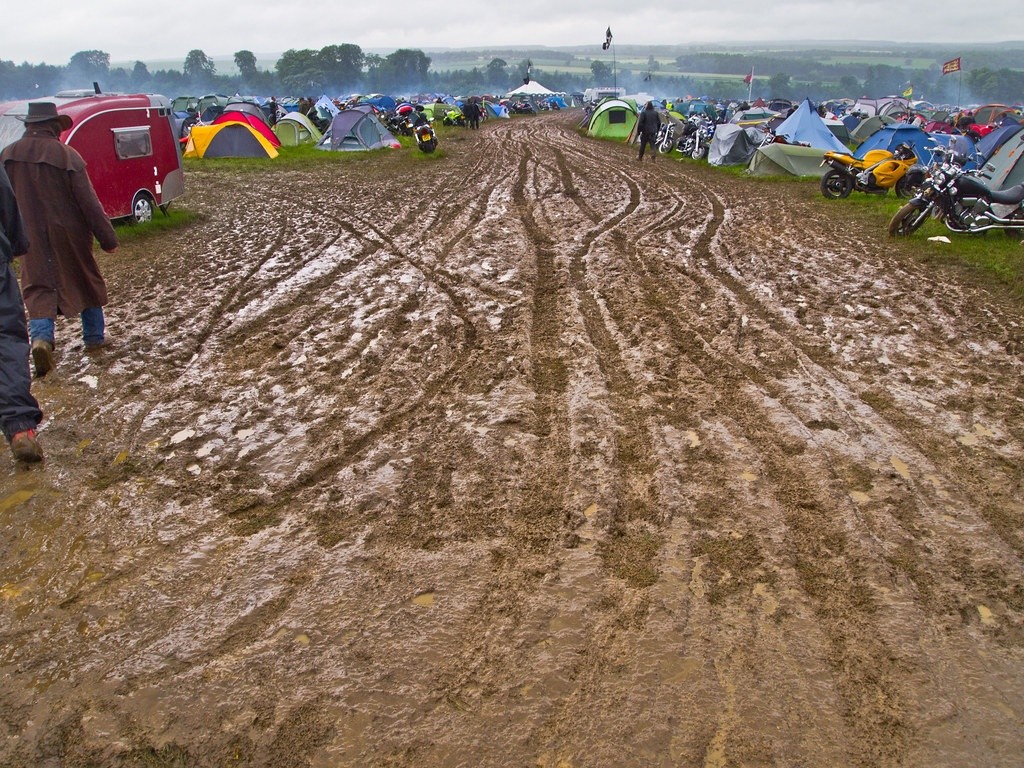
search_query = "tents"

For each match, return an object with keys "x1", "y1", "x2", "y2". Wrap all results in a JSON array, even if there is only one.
[
  {"x1": 569, "y1": 89, "x2": 1024, "y2": 202},
  {"x1": 162, "y1": 78, "x2": 571, "y2": 160}
]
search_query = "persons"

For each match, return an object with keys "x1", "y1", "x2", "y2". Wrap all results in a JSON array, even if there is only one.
[
  {"x1": 1, "y1": 102, "x2": 119, "y2": 378},
  {"x1": 0, "y1": 158, "x2": 47, "y2": 464}
]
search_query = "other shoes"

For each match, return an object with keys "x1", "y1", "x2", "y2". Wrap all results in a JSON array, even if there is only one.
[
  {"x1": 10, "y1": 431, "x2": 45, "y2": 462},
  {"x1": 31, "y1": 339, "x2": 57, "y2": 376},
  {"x1": 85, "y1": 337, "x2": 115, "y2": 349}
]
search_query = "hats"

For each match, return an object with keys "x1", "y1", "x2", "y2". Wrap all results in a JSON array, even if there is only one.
[{"x1": 15, "y1": 102, "x2": 74, "y2": 132}]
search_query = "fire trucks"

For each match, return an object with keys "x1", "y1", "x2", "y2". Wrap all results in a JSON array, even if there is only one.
[{"x1": 389, "y1": 105, "x2": 415, "y2": 137}]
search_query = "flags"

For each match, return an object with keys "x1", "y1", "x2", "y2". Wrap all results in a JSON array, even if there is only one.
[
  {"x1": 523, "y1": 61, "x2": 532, "y2": 85},
  {"x1": 601, "y1": 27, "x2": 612, "y2": 50},
  {"x1": 742, "y1": 69, "x2": 753, "y2": 86},
  {"x1": 942, "y1": 56, "x2": 961, "y2": 77},
  {"x1": 899, "y1": 80, "x2": 912, "y2": 99}
]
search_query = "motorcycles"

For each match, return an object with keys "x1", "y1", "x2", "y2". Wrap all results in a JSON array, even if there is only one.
[
  {"x1": 405, "y1": 105, "x2": 438, "y2": 152},
  {"x1": 639, "y1": 110, "x2": 677, "y2": 154},
  {"x1": 509, "y1": 102, "x2": 533, "y2": 114},
  {"x1": 818, "y1": 139, "x2": 925, "y2": 201},
  {"x1": 746, "y1": 123, "x2": 811, "y2": 168},
  {"x1": 676, "y1": 112, "x2": 721, "y2": 161},
  {"x1": 0, "y1": 90, "x2": 186, "y2": 226},
  {"x1": 441, "y1": 108, "x2": 465, "y2": 127},
  {"x1": 888, "y1": 138, "x2": 1024, "y2": 239},
  {"x1": 537, "y1": 100, "x2": 560, "y2": 111}
]
